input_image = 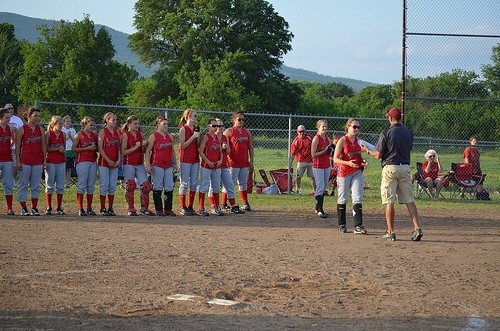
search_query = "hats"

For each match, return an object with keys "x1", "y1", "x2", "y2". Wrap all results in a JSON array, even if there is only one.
[{"x1": 385, "y1": 108, "x2": 401, "y2": 119}]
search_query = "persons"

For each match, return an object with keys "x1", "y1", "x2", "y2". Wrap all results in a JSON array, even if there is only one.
[
  {"x1": 208, "y1": 118, "x2": 245, "y2": 214},
  {"x1": 121, "y1": 115, "x2": 155, "y2": 216},
  {"x1": 0, "y1": 108, "x2": 16, "y2": 214},
  {"x1": 15, "y1": 108, "x2": 47, "y2": 216},
  {"x1": 179, "y1": 108, "x2": 200, "y2": 216},
  {"x1": 421, "y1": 149, "x2": 443, "y2": 201},
  {"x1": 311, "y1": 119, "x2": 336, "y2": 219},
  {"x1": 5, "y1": 103, "x2": 126, "y2": 188},
  {"x1": 360, "y1": 107, "x2": 423, "y2": 241},
  {"x1": 72, "y1": 116, "x2": 100, "y2": 216},
  {"x1": 198, "y1": 119, "x2": 225, "y2": 216},
  {"x1": 333, "y1": 118, "x2": 368, "y2": 235},
  {"x1": 145, "y1": 116, "x2": 177, "y2": 216},
  {"x1": 97, "y1": 112, "x2": 120, "y2": 216},
  {"x1": 221, "y1": 111, "x2": 254, "y2": 210},
  {"x1": 463, "y1": 134, "x2": 490, "y2": 201},
  {"x1": 290, "y1": 125, "x2": 367, "y2": 197},
  {"x1": 45, "y1": 116, "x2": 66, "y2": 215}
]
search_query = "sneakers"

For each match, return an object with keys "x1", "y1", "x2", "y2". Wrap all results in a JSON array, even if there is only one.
[
  {"x1": 316, "y1": 210, "x2": 327, "y2": 218},
  {"x1": 296, "y1": 189, "x2": 301, "y2": 195},
  {"x1": 156, "y1": 211, "x2": 167, "y2": 216},
  {"x1": 378, "y1": 231, "x2": 396, "y2": 241},
  {"x1": 86, "y1": 208, "x2": 97, "y2": 216},
  {"x1": 354, "y1": 225, "x2": 367, "y2": 235},
  {"x1": 179, "y1": 206, "x2": 192, "y2": 216},
  {"x1": 30, "y1": 208, "x2": 40, "y2": 216},
  {"x1": 198, "y1": 208, "x2": 209, "y2": 216},
  {"x1": 338, "y1": 224, "x2": 347, "y2": 233},
  {"x1": 162, "y1": 209, "x2": 177, "y2": 216},
  {"x1": 411, "y1": 229, "x2": 423, "y2": 240},
  {"x1": 128, "y1": 208, "x2": 137, "y2": 216},
  {"x1": 221, "y1": 205, "x2": 230, "y2": 209},
  {"x1": 210, "y1": 207, "x2": 224, "y2": 216},
  {"x1": 314, "y1": 210, "x2": 318, "y2": 214},
  {"x1": 107, "y1": 208, "x2": 116, "y2": 216},
  {"x1": 56, "y1": 208, "x2": 65, "y2": 215},
  {"x1": 8, "y1": 210, "x2": 15, "y2": 215},
  {"x1": 20, "y1": 206, "x2": 31, "y2": 216},
  {"x1": 98, "y1": 208, "x2": 109, "y2": 216},
  {"x1": 45, "y1": 208, "x2": 52, "y2": 215},
  {"x1": 79, "y1": 208, "x2": 87, "y2": 216},
  {"x1": 240, "y1": 203, "x2": 251, "y2": 211},
  {"x1": 230, "y1": 206, "x2": 246, "y2": 214},
  {"x1": 139, "y1": 209, "x2": 156, "y2": 216},
  {"x1": 187, "y1": 206, "x2": 197, "y2": 216}
]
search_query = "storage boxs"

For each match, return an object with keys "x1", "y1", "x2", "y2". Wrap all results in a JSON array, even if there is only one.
[{"x1": 269, "y1": 168, "x2": 294, "y2": 194}]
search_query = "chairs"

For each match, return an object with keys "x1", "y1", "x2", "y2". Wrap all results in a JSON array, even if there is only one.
[
  {"x1": 416, "y1": 162, "x2": 446, "y2": 200},
  {"x1": 449, "y1": 162, "x2": 487, "y2": 200}
]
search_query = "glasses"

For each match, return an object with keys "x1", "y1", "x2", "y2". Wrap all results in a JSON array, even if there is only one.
[
  {"x1": 8, "y1": 108, "x2": 13, "y2": 110},
  {"x1": 297, "y1": 130, "x2": 305, "y2": 133},
  {"x1": 387, "y1": 109, "x2": 390, "y2": 115},
  {"x1": 236, "y1": 118, "x2": 245, "y2": 122},
  {"x1": 218, "y1": 125, "x2": 225, "y2": 128},
  {"x1": 429, "y1": 155, "x2": 435, "y2": 158},
  {"x1": 349, "y1": 125, "x2": 361, "y2": 130},
  {"x1": 190, "y1": 114, "x2": 200, "y2": 118},
  {"x1": 209, "y1": 124, "x2": 219, "y2": 128}
]
